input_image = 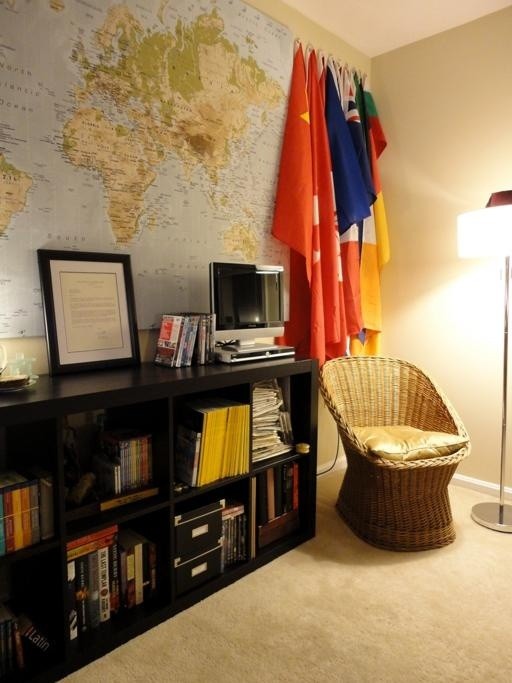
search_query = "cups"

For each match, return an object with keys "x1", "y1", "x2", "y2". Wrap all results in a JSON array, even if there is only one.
[
  {"x1": 0, "y1": 345, "x2": 8, "y2": 373},
  {"x1": 8, "y1": 357, "x2": 39, "y2": 386}
]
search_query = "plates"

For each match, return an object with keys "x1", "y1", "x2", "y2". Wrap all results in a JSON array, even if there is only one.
[{"x1": 0, "y1": 374, "x2": 31, "y2": 391}]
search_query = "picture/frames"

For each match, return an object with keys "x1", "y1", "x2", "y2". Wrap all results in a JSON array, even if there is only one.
[{"x1": 37, "y1": 249, "x2": 141, "y2": 376}]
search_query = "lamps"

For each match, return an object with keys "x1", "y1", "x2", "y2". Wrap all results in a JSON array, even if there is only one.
[{"x1": 471, "y1": 189, "x2": 512, "y2": 533}]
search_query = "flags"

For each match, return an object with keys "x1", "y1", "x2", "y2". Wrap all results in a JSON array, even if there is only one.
[{"x1": 269, "y1": 42, "x2": 391, "y2": 377}]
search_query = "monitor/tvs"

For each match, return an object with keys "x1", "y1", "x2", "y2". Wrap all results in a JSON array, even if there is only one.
[{"x1": 209, "y1": 262, "x2": 285, "y2": 350}]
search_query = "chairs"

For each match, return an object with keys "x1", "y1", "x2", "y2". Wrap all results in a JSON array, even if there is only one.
[{"x1": 317, "y1": 356, "x2": 471, "y2": 552}]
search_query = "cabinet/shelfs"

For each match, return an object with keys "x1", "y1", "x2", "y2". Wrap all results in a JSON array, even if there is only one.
[{"x1": 0, "y1": 358, "x2": 319, "y2": 682}]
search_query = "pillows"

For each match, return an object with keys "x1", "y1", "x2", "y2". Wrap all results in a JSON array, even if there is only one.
[{"x1": 348, "y1": 425, "x2": 468, "y2": 461}]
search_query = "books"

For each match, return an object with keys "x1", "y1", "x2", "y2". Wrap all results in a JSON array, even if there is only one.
[
  {"x1": 82, "y1": 430, "x2": 153, "y2": 495},
  {"x1": 174, "y1": 380, "x2": 299, "y2": 572},
  {"x1": 66, "y1": 524, "x2": 157, "y2": 640},
  {"x1": 154, "y1": 312, "x2": 217, "y2": 369},
  {"x1": 0, "y1": 472, "x2": 54, "y2": 557},
  {"x1": 0, "y1": 606, "x2": 49, "y2": 676}
]
